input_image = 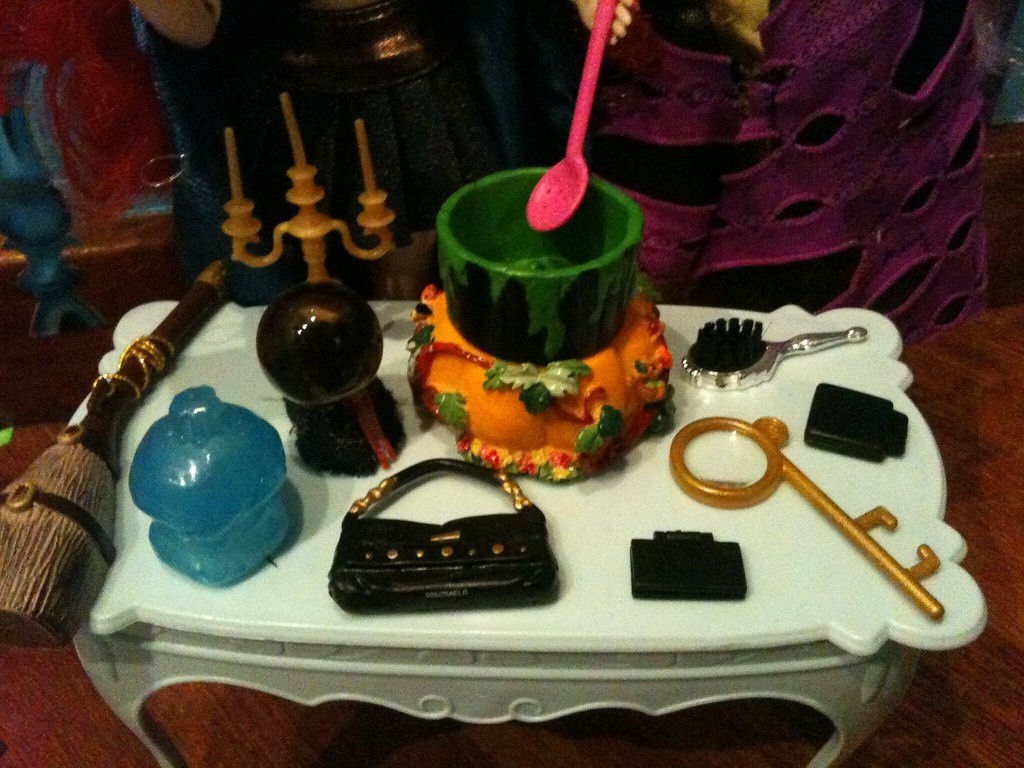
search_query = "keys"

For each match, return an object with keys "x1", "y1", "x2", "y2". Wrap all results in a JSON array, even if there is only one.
[{"x1": 673, "y1": 414, "x2": 945, "y2": 623}]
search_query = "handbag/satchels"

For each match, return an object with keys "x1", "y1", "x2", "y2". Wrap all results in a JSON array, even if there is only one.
[{"x1": 324, "y1": 459, "x2": 558, "y2": 613}]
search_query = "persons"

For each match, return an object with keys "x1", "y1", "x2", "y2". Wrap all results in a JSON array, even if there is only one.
[{"x1": 130, "y1": 0, "x2": 1015, "y2": 340}]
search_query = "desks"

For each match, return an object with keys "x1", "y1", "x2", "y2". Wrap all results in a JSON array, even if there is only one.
[{"x1": 73, "y1": 300, "x2": 988, "y2": 768}]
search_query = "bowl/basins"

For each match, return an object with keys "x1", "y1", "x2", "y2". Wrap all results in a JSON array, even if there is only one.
[{"x1": 436, "y1": 166, "x2": 644, "y2": 366}]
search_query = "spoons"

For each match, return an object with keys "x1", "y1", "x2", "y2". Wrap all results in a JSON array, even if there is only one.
[{"x1": 526, "y1": 0, "x2": 615, "y2": 229}]
